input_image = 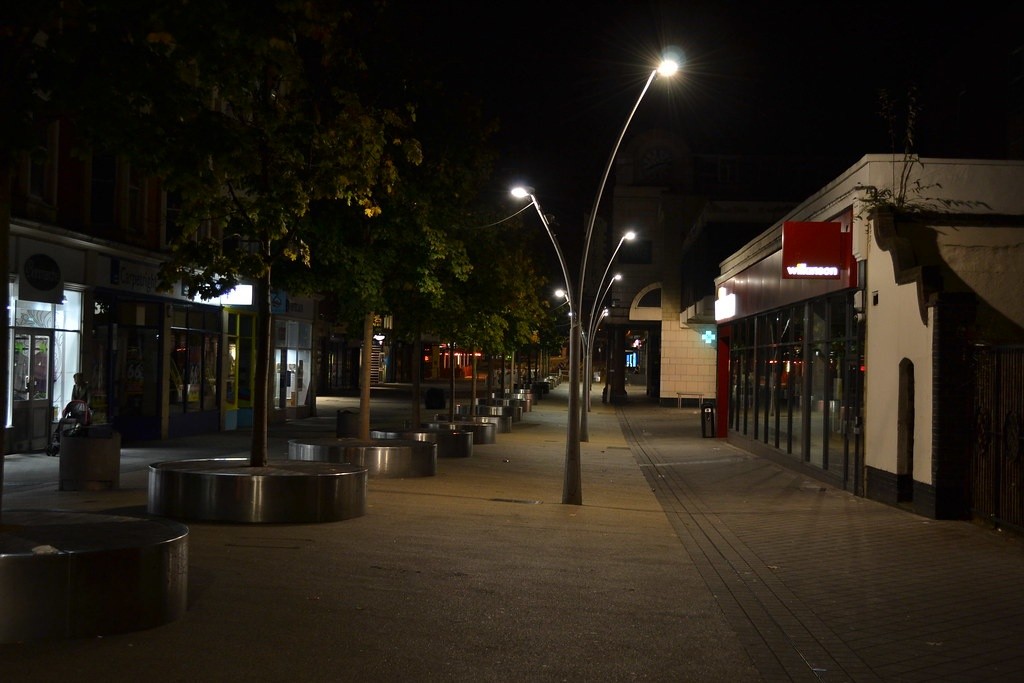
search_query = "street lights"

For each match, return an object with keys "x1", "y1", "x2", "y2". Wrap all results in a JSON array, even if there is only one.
[
  {"x1": 552, "y1": 231, "x2": 642, "y2": 441},
  {"x1": 504, "y1": 45, "x2": 681, "y2": 510}
]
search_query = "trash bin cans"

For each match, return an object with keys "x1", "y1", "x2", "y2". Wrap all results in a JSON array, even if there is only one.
[
  {"x1": 59, "y1": 425, "x2": 122, "y2": 490},
  {"x1": 336, "y1": 407, "x2": 361, "y2": 439},
  {"x1": 701, "y1": 403, "x2": 714, "y2": 438},
  {"x1": 425, "y1": 387, "x2": 446, "y2": 409},
  {"x1": 540, "y1": 382, "x2": 550, "y2": 394}
]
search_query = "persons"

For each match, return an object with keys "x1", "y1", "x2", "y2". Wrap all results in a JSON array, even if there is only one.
[{"x1": 72, "y1": 373, "x2": 91, "y2": 405}]
